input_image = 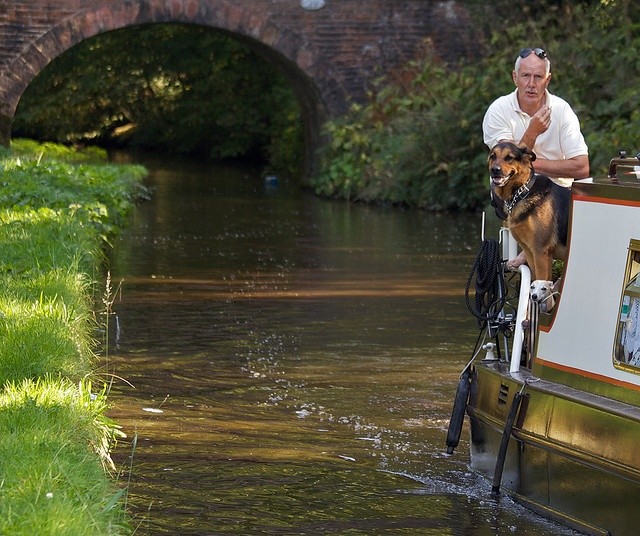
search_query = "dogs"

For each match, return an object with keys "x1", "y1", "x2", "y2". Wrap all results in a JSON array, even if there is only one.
[
  {"x1": 529, "y1": 280, "x2": 555, "y2": 315},
  {"x1": 486, "y1": 142, "x2": 572, "y2": 279}
]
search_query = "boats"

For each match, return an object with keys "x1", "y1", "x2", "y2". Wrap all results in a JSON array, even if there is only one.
[{"x1": 464, "y1": 151, "x2": 640, "y2": 536}]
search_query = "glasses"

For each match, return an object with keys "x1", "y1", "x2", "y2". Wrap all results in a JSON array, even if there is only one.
[{"x1": 520, "y1": 47, "x2": 547, "y2": 59}]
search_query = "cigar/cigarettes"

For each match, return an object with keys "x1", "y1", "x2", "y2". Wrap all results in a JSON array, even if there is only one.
[{"x1": 547, "y1": 105, "x2": 553, "y2": 111}]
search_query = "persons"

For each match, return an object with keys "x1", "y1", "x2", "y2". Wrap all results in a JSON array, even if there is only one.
[{"x1": 482, "y1": 48, "x2": 590, "y2": 268}]
